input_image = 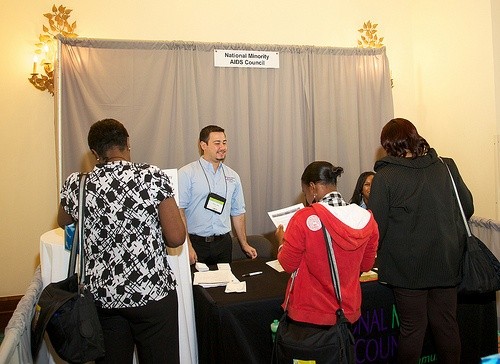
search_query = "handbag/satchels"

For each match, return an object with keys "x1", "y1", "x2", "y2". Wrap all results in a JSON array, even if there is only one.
[
  {"x1": 460, "y1": 233, "x2": 500, "y2": 292},
  {"x1": 271, "y1": 308, "x2": 355, "y2": 364},
  {"x1": 30, "y1": 273, "x2": 105, "y2": 364}
]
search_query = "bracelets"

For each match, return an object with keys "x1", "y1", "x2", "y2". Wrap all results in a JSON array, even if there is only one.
[{"x1": 278, "y1": 245, "x2": 283, "y2": 251}]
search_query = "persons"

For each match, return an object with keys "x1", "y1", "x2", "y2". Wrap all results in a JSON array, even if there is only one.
[
  {"x1": 276, "y1": 161, "x2": 379, "y2": 328},
  {"x1": 348, "y1": 172, "x2": 376, "y2": 210},
  {"x1": 57, "y1": 119, "x2": 186, "y2": 364},
  {"x1": 366, "y1": 118, "x2": 474, "y2": 364},
  {"x1": 177, "y1": 124, "x2": 257, "y2": 267}
]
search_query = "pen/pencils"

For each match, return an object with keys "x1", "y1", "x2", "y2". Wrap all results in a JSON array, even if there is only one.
[{"x1": 242, "y1": 271, "x2": 263, "y2": 277}]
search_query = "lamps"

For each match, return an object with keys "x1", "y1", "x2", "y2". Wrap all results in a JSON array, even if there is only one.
[
  {"x1": 356, "y1": 20, "x2": 395, "y2": 102},
  {"x1": 29, "y1": 3, "x2": 79, "y2": 97}
]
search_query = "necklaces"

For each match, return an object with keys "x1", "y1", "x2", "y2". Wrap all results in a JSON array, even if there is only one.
[{"x1": 106, "y1": 157, "x2": 127, "y2": 161}]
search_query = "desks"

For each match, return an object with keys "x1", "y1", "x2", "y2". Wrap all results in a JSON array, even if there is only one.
[
  {"x1": 193, "y1": 257, "x2": 498, "y2": 364},
  {"x1": 39, "y1": 228, "x2": 199, "y2": 364}
]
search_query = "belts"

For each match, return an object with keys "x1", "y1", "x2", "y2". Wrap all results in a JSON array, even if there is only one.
[{"x1": 191, "y1": 235, "x2": 226, "y2": 243}]
